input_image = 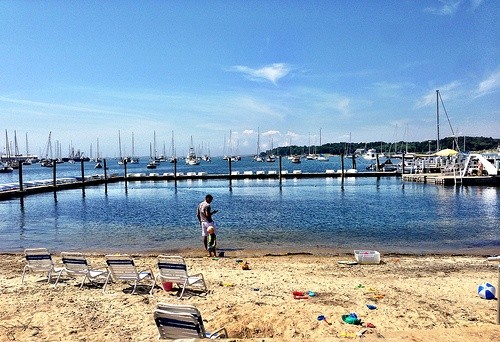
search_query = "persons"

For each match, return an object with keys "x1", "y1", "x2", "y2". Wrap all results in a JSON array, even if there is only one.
[
  {"x1": 197, "y1": 195, "x2": 217, "y2": 249},
  {"x1": 207, "y1": 226, "x2": 216, "y2": 257},
  {"x1": 352, "y1": 152, "x2": 356, "y2": 169}
]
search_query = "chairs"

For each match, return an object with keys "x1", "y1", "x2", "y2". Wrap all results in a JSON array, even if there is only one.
[
  {"x1": 21, "y1": 249, "x2": 67, "y2": 287},
  {"x1": 148, "y1": 255, "x2": 209, "y2": 298},
  {"x1": 154, "y1": 303, "x2": 228, "y2": 339},
  {"x1": 54, "y1": 252, "x2": 116, "y2": 291},
  {"x1": 100, "y1": 254, "x2": 157, "y2": 295}
]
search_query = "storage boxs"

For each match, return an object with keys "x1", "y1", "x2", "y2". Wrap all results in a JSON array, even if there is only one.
[{"x1": 354, "y1": 250, "x2": 380, "y2": 265}]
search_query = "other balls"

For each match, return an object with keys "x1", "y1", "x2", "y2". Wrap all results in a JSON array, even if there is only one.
[{"x1": 478, "y1": 283, "x2": 495, "y2": 299}]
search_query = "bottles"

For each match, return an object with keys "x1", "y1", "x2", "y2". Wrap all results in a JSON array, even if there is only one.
[{"x1": 293, "y1": 292, "x2": 304, "y2": 296}]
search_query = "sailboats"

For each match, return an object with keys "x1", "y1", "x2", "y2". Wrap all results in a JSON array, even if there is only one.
[{"x1": 1, "y1": 89, "x2": 470, "y2": 173}]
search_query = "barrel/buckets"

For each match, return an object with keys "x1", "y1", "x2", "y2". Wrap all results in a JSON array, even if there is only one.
[{"x1": 162, "y1": 282, "x2": 172, "y2": 291}]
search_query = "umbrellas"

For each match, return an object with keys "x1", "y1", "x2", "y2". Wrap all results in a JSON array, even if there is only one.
[{"x1": 431, "y1": 148, "x2": 459, "y2": 160}]
